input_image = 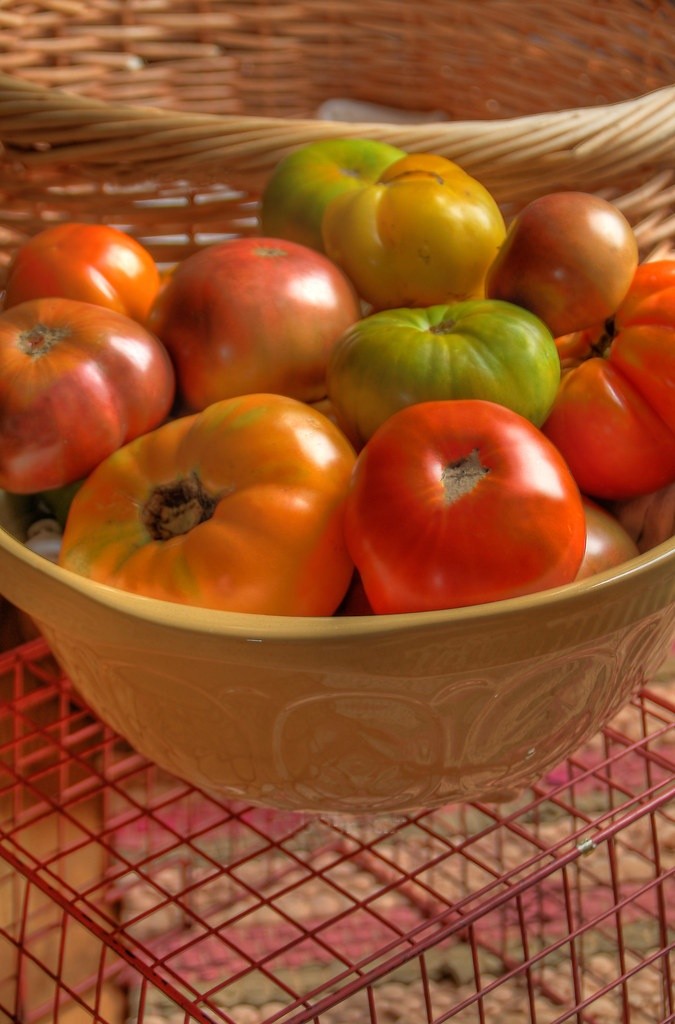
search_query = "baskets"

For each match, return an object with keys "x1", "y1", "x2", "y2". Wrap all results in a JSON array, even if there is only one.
[{"x1": 0, "y1": 0, "x2": 675, "y2": 319}]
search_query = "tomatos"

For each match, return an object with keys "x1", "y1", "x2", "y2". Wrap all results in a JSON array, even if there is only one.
[{"x1": 0, "y1": 138, "x2": 674, "y2": 619}]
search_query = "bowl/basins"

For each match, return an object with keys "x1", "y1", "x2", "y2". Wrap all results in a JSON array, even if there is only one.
[{"x1": 0, "y1": 483, "x2": 675, "y2": 807}]
